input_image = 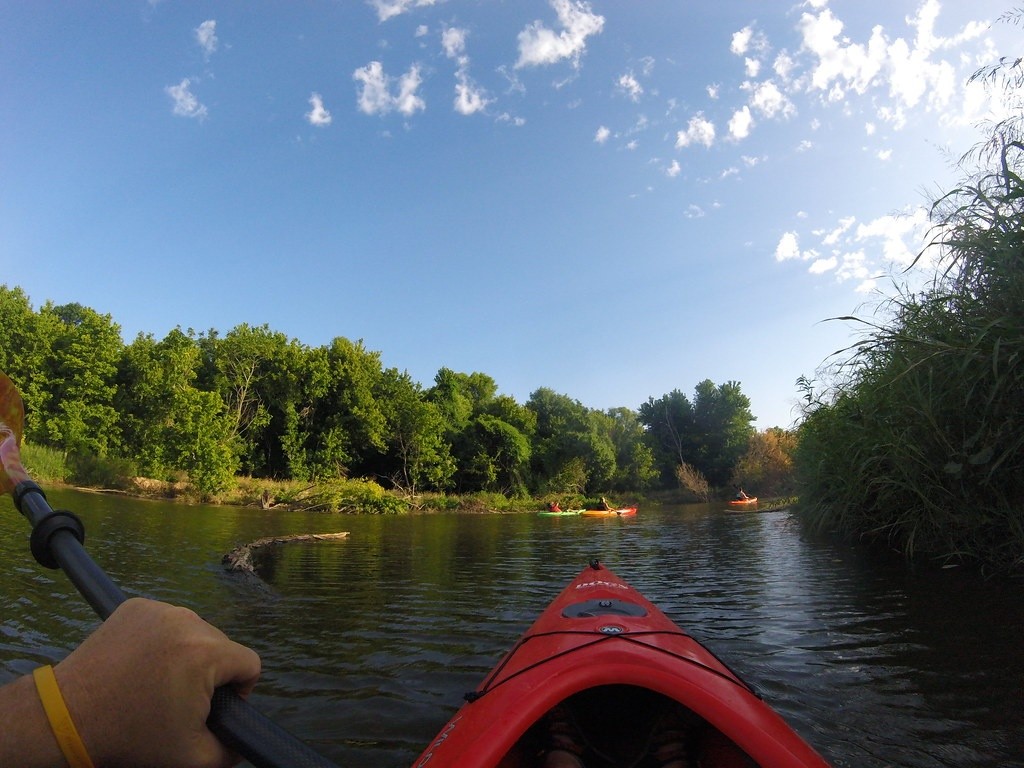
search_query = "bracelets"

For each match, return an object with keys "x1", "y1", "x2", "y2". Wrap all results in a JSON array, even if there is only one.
[{"x1": 32, "y1": 663, "x2": 91, "y2": 767}]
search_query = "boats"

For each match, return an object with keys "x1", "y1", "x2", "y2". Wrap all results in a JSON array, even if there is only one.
[
  {"x1": 579, "y1": 506, "x2": 638, "y2": 517},
  {"x1": 537, "y1": 508, "x2": 587, "y2": 517},
  {"x1": 728, "y1": 496, "x2": 758, "y2": 505}
]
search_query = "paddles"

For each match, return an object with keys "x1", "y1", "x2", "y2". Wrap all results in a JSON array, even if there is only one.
[{"x1": 0, "y1": 368, "x2": 342, "y2": 768}]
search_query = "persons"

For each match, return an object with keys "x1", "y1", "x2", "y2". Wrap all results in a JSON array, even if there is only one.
[
  {"x1": 736, "y1": 487, "x2": 750, "y2": 501},
  {"x1": 547, "y1": 502, "x2": 564, "y2": 512},
  {"x1": 597, "y1": 496, "x2": 611, "y2": 510},
  {"x1": 1, "y1": 597, "x2": 261, "y2": 768}
]
